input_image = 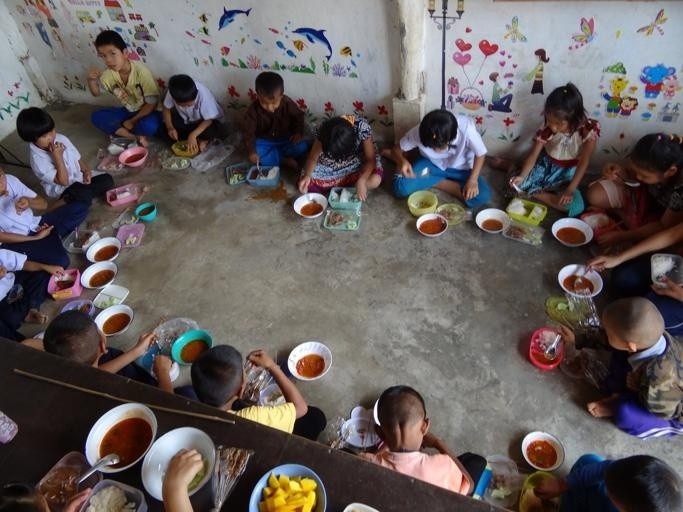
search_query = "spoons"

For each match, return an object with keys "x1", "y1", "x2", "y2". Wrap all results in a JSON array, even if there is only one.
[
  {"x1": 510, "y1": 179, "x2": 531, "y2": 199},
  {"x1": 609, "y1": 167, "x2": 641, "y2": 188},
  {"x1": 76, "y1": 454, "x2": 120, "y2": 484},
  {"x1": 306, "y1": 192, "x2": 317, "y2": 204},
  {"x1": 256, "y1": 162, "x2": 264, "y2": 176},
  {"x1": 574, "y1": 263, "x2": 591, "y2": 290},
  {"x1": 411, "y1": 167, "x2": 430, "y2": 180},
  {"x1": 545, "y1": 332, "x2": 561, "y2": 361}
]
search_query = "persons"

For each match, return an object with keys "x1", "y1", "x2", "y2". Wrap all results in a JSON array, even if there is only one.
[
  {"x1": 88, "y1": 28, "x2": 163, "y2": 148},
  {"x1": 495, "y1": 80, "x2": 600, "y2": 218},
  {"x1": 2, "y1": 309, "x2": 681, "y2": 510},
  {"x1": 241, "y1": 70, "x2": 311, "y2": 170},
  {"x1": 579, "y1": 131, "x2": 683, "y2": 253},
  {"x1": 585, "y1": 221, "x2": 683, "y2": 336},
  {"x1": 586, "y1": 296, "x2": 682, "y2": 440},
  {"x1": 300, "y1": 114, "x2": 383, "y2": 204},
  {"x1": 163, "y1": 74, "x2": 225, "y2": 153},
  {"x1": 388, "y1": 109, "x2": 493, "y2": 211},
  {"x1": 1, "y1": 108, "x2": 116, "y2": 335}
]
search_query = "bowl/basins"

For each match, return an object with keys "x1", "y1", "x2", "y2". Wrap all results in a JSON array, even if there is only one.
[
  {"x1": 172, "y1": 331, "x2": 212, "y2": 364},
  {"x1": 118, "y1": 147, "x2": 148, "y2": 169},
  {"x1": 551, "y1": 218, "x2": 593, "y2": 249},
  {"x1": 557, "y1": 264, "x2": 603, "y2": 298},
  {"x1": 83, "y1": 401, "x2": 327, "y2": 512},
  {"x1": 521, "y1": 431, "x2": 565, "y2": 472},
  {"x1": 407, "y1": 191, "x2": 511, "y2": 237},
  {"x1": 294, "y1": 193, "x2": 327, "y2": 219},
  {"x1": 80, "y1": 202, "x2": 159, "y2": 336},
  {"x1": 286, "y1": 341, "x2": 332, "y2": 381}
]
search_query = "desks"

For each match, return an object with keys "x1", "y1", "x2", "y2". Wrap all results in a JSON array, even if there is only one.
[{"x1": 0, "y1": 337, "x2": 510, "y2": 512}]
